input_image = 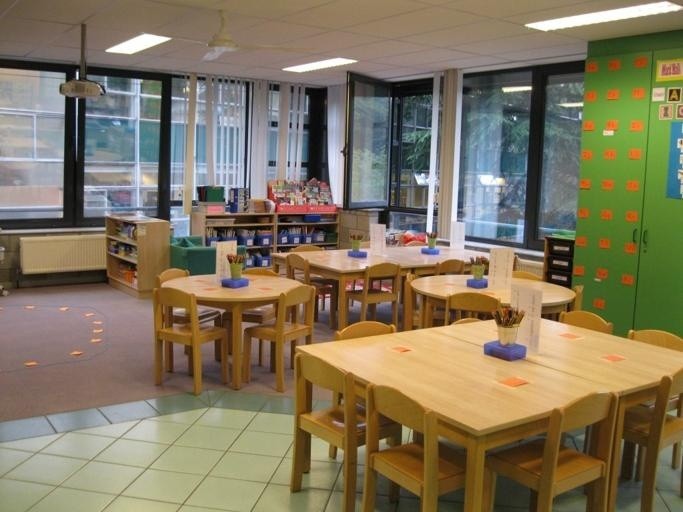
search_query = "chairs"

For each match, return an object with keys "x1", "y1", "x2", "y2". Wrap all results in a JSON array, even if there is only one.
[
  {"x1": 361, "y1": 383, "x2": 466, "y2": 511},
  {"x1": 559, "y1": 310, "x2": 613, "y2": 333},
  {"x1": 483, "y1": 392, "x2": 619, "y2": 511},
  {"x1": 290, "y1": 351, "x2": 402, "y2": 512},
  {"x1": 584, "y1": 329, "x2": 683, "y2": 482},
  {"x1": 223, "y1": 267, "x2": 280, "y2": 355},
  {"x1": 268, "y1": 246, "x2": 543, "y2": 339},
  {"x1": 153, "y1": 287, "x2": 228, "y2": 395},
  {"x1": 585, "y1": 368, "x2": 683, "y2": 512},
  {"x1": 155, "y1": 268, "x2": 222, "y2": 363},
  {"x1": 242, "y1": 285, "x2": 316, "y2": 392}
]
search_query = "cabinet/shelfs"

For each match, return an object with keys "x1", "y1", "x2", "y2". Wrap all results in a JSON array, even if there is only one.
[
  {"x1": 105, "y1": 215, "x2": 170, "y2": 299},
  {"x1": 572, "y1": 29, "x2": 683, "y2": 341},
  {"x1": 543, "y1": 237, "x2": 574, "y2": 288},
  {"x1": 275, "y1": 211, "x2": 340, "y2": 252},
  {"x1": 191, "y1": 212, "x2": 276, "y2": 270}
]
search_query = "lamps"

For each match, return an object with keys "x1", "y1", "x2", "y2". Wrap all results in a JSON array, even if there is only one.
[{"x1": 60, "y1": 23, "x2": 105, "y2": 98}]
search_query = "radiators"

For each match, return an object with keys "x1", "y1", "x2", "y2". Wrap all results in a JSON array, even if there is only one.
[{"x1": 17, "y1": 234, "x2": 107, "y2": 275}]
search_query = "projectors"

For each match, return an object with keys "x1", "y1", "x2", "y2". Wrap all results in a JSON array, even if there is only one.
[{"x1": 58, "y1": 79, "x2": 105, "y2": 97}]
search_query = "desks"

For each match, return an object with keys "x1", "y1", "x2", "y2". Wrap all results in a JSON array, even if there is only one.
[
  {"x1": 295, "y1": 326, "x2": 614, "y2": 512},
  {"x1": 435, "y1": 317, "x2": 683, "y2": 511},
  {"x1": 162, "y1": 274, "x2": 302, "y2": 390}
]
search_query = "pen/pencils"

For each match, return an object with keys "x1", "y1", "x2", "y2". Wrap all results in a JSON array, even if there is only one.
[
  {"x1": 227, "y1": 254, "x2": 243, "y2": 264},
  {"x1": 470, "y1": 257, "x2": 483, "y2": 265},
  {"x1": 426, "y1": 231, "x2": 438, "y2": 238},
  {"x1": 352, "y1": 234, "x2": 363, "y2": 240},
  {"x1": 493, "y1": 302, "x2": 525, "y2": 328}
]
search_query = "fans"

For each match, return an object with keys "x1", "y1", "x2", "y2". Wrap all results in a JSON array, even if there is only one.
[{"x1": 136, "y1": 7, "x2": 311, "y2": 64}]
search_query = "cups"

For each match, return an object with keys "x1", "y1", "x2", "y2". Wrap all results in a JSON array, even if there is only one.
[
  {"x1": 230, "y1": 263, "x2": 242, "y2": 279},
  {"x1": 472, "y1": 264, "x2": 484, "y2": 280},
  {"x1": 498, "y1": 327, "x2": 518, "y2": 347},
  {"x1": 352, "y1": 240, "x2": 361, "y2": 252},
  {"x1": 428, "y1": 238, "x2": 436, "y2": 249}
]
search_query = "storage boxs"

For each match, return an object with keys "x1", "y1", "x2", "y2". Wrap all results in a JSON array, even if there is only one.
[
  {"x1": 243, "y1": 256, "x2": 255, "y2": 268},
  {"x1": 198, "y1": 202, "x2": 225, "y2": 213},
  {"x1": 313, "y1": 233, "x2": 324, "y2": 243},
  {"x1": 277, "y1": 234, "x2": 290, "y2": 244},
  {"x1": 219, "y1": 237, "x2": 237, "y2": 241},
  {"x1": 255, "y1": 256, "x2": 272, "y2": 267},
  {"x1": 237, "y1": 236, "x2": 255, "y2": 247},
  {"x1": 206, "y1": 238, "x2": 219, "y2": 246},
  {"x1": 290, "y1": 234, "x2": 304, "y2": 244},
  {"x1": 255, "y1": 235, "x2": 272, "y2": 246},
  {"x1": 303, "y1": 235, "x2": 313, "y2": 244},
  {"x1": 325, "y1": 232, "x2": 338, "y2": 243}
]
